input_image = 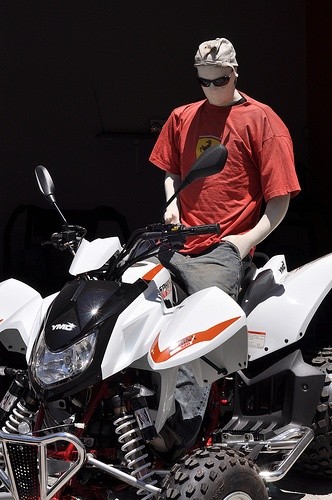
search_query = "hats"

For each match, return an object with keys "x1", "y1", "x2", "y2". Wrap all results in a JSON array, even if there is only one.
[{"x1": 194, "y1": 39, "x2": 239, "y2": 78}]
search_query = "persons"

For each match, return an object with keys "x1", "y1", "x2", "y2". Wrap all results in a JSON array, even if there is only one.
[{"x1": 138, "y1": 37, "x2": 301, "y2": 464}]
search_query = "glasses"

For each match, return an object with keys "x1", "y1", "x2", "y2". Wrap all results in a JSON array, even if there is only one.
[{"x1": 197, "y1": 67, "x2": 234, "y2": 88}]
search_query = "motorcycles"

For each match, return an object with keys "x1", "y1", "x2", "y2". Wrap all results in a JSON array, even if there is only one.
[{"x1": 0, "y1": 146, "x2": 332, "y2": 500}]
